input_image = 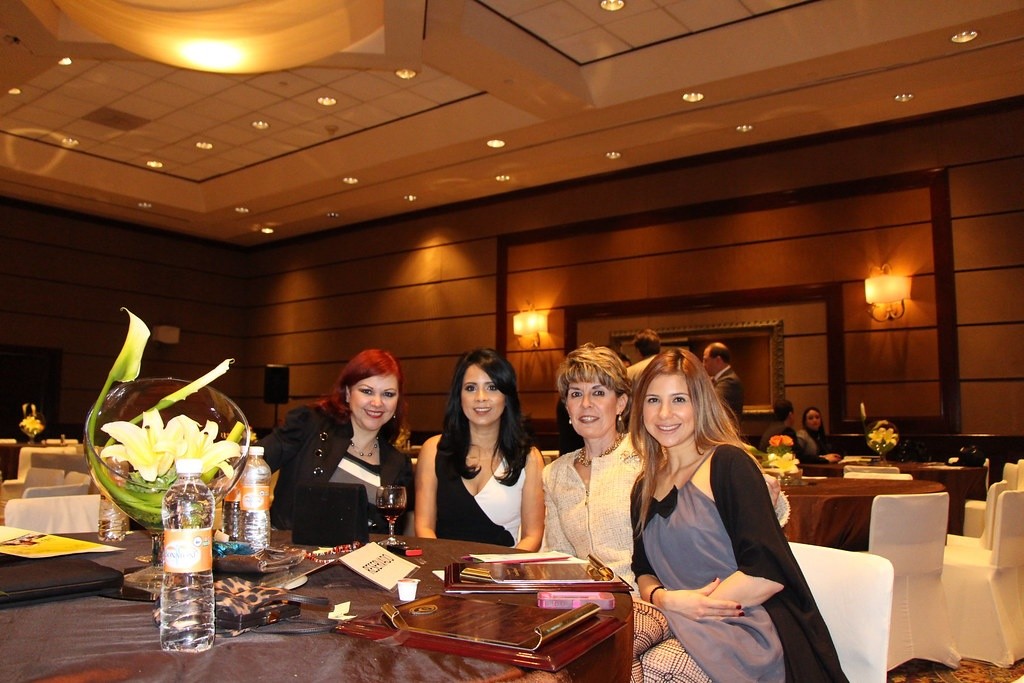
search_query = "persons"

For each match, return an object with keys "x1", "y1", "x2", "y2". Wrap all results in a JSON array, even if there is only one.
[
  {"x1": 630, "y1": 348, "x2": 850, "y2": 683},
  {"x1": 542, "y1": 342, "x2": 790, "y2": 598},
  {"x1": 795, "y1": 406, "x2": 829, "y2": 456},
  {"x1": 703, "y1": 343, "x2": 743, "y2": 425},
  {"x1": 251, "y1": 348, "x2": 414, "y2": 547},
  {"x1": 626, "y1": 329, "x2": 660, "y2": 386},
  {"x1": 617, "y1": 352, "x2": 632, "y2": 367},
  {"x1": 555, "y1": 399, "x2": 585, "y2": 457},
  {"x1": 414, "y1": 347, "x2": 546, "y2": 553},
  {"x1": 757, "y1": 399, "x2": 841, "y2": 464}
]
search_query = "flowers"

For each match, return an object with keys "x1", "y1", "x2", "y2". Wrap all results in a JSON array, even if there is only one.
[
  {"x1": 21, "y1": 402, "x2": 43, "y2": 434},
  {"x1": 867, "y1": 421, "x2": 898, "y2": 448},
  {"x1": 764, "y1": 433, "x2": 800, "y2": 476},
  {"x1": 86, "y1": 302, "x2": 241, "y2": 510}
]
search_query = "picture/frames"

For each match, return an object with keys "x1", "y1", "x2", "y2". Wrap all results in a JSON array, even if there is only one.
[{"x1": 607, "y1": 318, "x2": 786, "y2": 421}]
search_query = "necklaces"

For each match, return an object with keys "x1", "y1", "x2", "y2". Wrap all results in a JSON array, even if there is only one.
[
  {"x1": 578, "y1": 433, "x2": 624, "y2": 467},
  {"x1": 351, "y1": 437, "x2": 378, "y2": 456}
]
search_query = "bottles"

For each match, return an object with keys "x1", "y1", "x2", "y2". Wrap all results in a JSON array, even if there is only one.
[
  {"x1": 160, "y1": 459, "x2": 215, "y2": 654},
  {"x1": 98, "y1": 457, "x2": 129, "y2": 542},
  {"x1": 222, "y1": 445, "x2": 271, "y2": 552}
]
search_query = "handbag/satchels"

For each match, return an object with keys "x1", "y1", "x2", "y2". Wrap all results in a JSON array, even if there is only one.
[
  {"x1": 151, "y1": 576, "x2": 337, "y2": 638},
  {"x1": 292, "y1": 480, "x2": 370, "y2": 546}
]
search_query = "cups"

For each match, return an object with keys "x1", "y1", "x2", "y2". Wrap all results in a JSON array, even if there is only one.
[{"x1": 396, "y1": 578, "x2": 421, "y2": 601}]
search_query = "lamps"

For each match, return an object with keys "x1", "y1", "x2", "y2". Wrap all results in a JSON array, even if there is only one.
[
  {"x1": 265, "y1": 364, "x2": 289, "y2": 428},
  {"x1": 513, "y1": 299, "x2": 547, "y2": 349},
  {"x1": 52, "y1": 0, "x2": 384, "y2": 75},
  {"x1": 152, "y1": 319, "x2": 180, "y2": 363},
  {"x1": 864, "y1": 263, "x2": 912, "y2": 322}
]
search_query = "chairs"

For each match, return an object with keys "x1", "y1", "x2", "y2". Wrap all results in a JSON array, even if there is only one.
[
  {"x1": 786, "y1": 542, "x2": 895, "y2": 683},
  {"x1": 802, "y1": 455, "x2": 914, "y2": 480},
  {"x1": 939, "y1": 458, "x2": 1024, "y2": 669},
  {"x1": 0, "y1": 439, "x2": 130, "y2": 543},
  {"x1": 868, "y1": 493, "x2": 963, "y2": 671}
]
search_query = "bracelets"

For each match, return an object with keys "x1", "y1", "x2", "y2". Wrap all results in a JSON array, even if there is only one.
[{"x1": 650, "y1": 586, "x2": 667, "y2": 604}]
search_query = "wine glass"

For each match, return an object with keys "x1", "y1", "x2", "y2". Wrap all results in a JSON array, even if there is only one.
[{"x1": 376, "y1": 486, "x2": 406, "y2": 546}]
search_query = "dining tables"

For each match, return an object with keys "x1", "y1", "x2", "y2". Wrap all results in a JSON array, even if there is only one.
[
  {"x1": 795, "y1": 461, "x2": 988, "y2": 536},
  {"x1": 0, "y1": 442, "x2": 69, "y2": 483},
  {"x1": 766, "y1": 471, "x2": 950, "y2": 551},
  {"x1": 18, "y1": 446, "x2": 79, "y2": 483},
  {"x1": 0, "y1": 528, "x2": 635, "y2": 683}
]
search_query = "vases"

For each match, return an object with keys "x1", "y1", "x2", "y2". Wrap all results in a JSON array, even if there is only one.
[
  {"x1": 19, "y1": 413, "x2": 46, "y2": 445},
  {"x1": 863, "y1": 420, "x2": 900, "y2": 466},
  {"x1": 83, "y1": 376, "x2": 253, "y2": 602},
  {"x1": 761, "y1": 454, "x2": 802, "y2": 486}
]
